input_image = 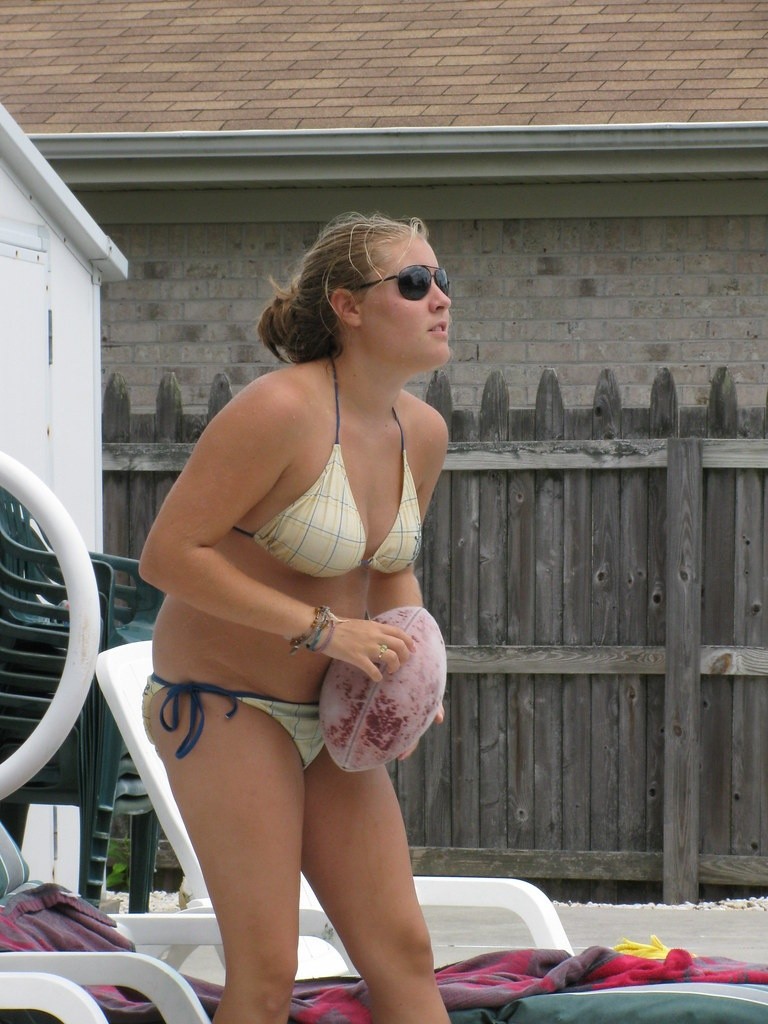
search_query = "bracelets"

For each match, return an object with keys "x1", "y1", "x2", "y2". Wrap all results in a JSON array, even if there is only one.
[{"x1": 288, "y1": 602, "x2": 338, "y2": 654}]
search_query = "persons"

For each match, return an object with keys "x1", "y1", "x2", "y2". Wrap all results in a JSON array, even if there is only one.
[{"x1": 133, "y1": 216, "x2": 460, "y2": 1024}]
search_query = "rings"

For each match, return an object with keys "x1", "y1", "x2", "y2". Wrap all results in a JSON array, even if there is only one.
[{"x1": 378, "y1": 644, "x2": 388, "y2": 664}]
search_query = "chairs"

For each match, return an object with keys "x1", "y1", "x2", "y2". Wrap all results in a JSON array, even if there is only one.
[
  {"x1": 97, "y1": 639, "x2": 576, "y2": 963},
  {"x1": 0, "y1": 486, "x2": 169, "y2": 910},
  {"x1": 2, "y1": 822, "x2": 768, "y2": 1024}
]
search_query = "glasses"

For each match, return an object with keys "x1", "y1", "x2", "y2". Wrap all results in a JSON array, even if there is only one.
[{"x1": 343, "y1": 266, "x2": 449, "y2": 301}]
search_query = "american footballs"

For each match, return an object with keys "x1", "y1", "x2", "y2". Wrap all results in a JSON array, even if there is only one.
[{"x1": 319, "y1": 606, "x2": 447, "y2": 771}]
